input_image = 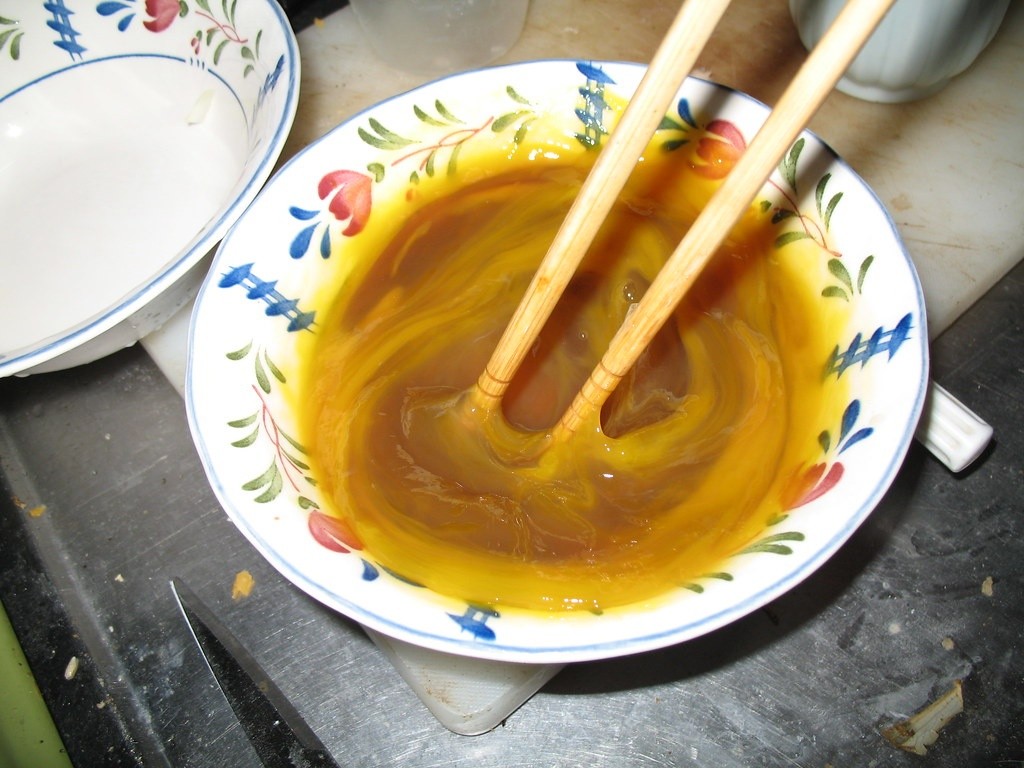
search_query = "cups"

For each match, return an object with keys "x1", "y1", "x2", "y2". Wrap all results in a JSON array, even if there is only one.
[{"x1": 789, "y1": 0, "x2": 1009, "y2": 104}]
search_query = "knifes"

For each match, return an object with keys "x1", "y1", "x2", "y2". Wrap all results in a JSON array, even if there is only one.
[{"x1": 168, "y1": 577, "x2": 342, "y2": 768}]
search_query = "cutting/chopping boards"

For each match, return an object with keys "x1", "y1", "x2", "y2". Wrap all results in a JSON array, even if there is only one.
[{"x1": 140, "y1": 1, "x2": 1024, "y2": 737}]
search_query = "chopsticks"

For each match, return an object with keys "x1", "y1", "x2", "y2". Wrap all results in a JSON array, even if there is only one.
[{"x1": 460, "y1": 0, "x2": 896, "y2": 445}]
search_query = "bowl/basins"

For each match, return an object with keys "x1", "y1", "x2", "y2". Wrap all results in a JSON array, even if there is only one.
[
  {"x1": 0, "y1": 0, "x2": 300, "y2": 378},
  {"x1": 184, "y1": 62, "x2": 929, "y2": 665}
]
